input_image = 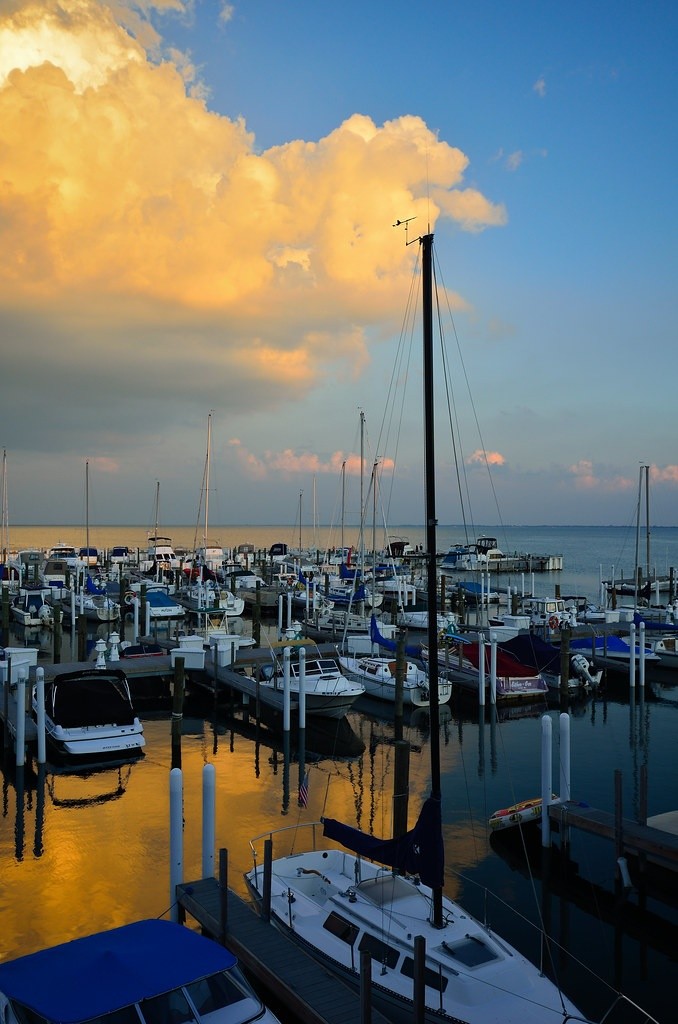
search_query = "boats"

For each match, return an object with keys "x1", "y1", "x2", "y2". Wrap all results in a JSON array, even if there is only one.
[
  {"x1": 0, "y1": 407, "x2": 678, "y2": 782},
  {"x1": 248, "y1": 212, "x2": 594, "y2": 1024},
  {"x1": 2, "y1": 918, "x2": 277, "y2": 1024}
]
